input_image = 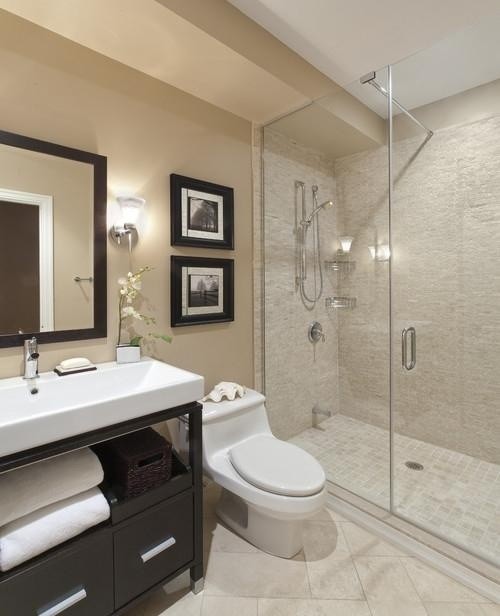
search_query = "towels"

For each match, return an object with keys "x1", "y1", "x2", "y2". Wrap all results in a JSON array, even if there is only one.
[{"x1": 1, "y1": 446, "x2": 111, "y2": 573}]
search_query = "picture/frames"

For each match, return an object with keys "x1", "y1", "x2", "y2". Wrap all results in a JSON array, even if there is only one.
[
  {"x1": 169, "y1": 173, "x2": 235, "y2": 252},
  {"x1": 170, "y1": 255, "x2": 235, "y2": 328}
]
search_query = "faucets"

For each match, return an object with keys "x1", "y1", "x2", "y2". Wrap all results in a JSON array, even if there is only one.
[{"x1": 23, "y1": 338, "x2": 40, "y2": 378}]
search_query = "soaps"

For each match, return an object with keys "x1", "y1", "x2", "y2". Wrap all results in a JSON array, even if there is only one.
[{"x1": 60, "y1": 357, "x2": 92, "y2": 369}]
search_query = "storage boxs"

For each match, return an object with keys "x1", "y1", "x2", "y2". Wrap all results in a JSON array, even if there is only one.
[{"x1": 114, "y1": 431, "x2": 173, "y2": 503}]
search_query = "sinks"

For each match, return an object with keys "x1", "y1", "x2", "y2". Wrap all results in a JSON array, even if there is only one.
[{"x1": 0, "y1": 359, "x2": 205, "y2": 456}]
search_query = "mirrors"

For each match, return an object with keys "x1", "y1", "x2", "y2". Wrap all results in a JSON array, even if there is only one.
[{"x1": 0, "y1": 131, "x2": 108, "y2": 352}]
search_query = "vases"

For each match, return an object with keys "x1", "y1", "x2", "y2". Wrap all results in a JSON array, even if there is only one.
[{"x1": 116, "y1": 346, "x2": 141, "y2": 362}]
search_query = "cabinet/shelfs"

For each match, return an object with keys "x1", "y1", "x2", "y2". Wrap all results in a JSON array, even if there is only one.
[{"x1": 0, "y1": 401, "x2": 205, "y2": 616}]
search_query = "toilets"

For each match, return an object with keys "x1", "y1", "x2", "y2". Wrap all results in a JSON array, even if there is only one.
[{"x1": 167, "y1": 385, "x2": 329, "y2": 560}]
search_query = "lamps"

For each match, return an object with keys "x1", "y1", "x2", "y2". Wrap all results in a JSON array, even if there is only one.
[
  {"x1": 338, "y1": 235, "x2": 354, "y2": 257},
  {"x1": 109, "y1": 193, "x2": 146, "y2": 251}
]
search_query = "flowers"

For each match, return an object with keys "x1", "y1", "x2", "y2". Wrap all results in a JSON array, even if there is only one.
[{"x1": 116, "y1": 262, "x2": 172, "y2": 347}]
